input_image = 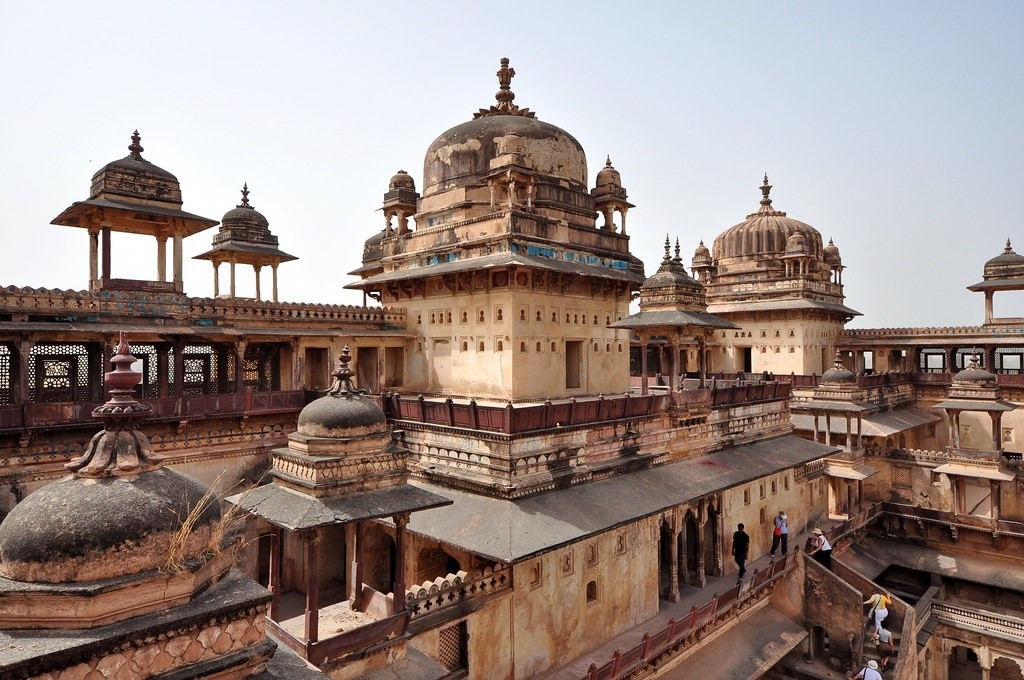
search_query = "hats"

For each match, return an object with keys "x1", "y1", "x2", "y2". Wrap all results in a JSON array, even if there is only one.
[
  {"x1": 868, "y1": 660, "x2": 878, "y2": 669},
  {"x1": 812, "y1": 528, "x2": 822, "y2": 535},
  {"x1": 780, "y1": 511, "x2": 787, "y2": 519}
]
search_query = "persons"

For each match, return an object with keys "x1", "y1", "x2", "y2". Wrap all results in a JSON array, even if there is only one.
[
  {"x1": 871, "y1": 620, "x2": 894, "y2": 671},
  {"x1": 769, "y1": 510, "x2": 788, "y2": 557},
  {"x1": 732, "y1": 523, "x2": 750, "y2": 578},
  {"x1": 808, "y1": 527, "x2": 833, "y2": 570},
  {"x1": 863, "y1": 587, "x2": 892, "y2": 633},
  {"x1": 848, "y1": 660, "x2": 883, "y2": 680}
]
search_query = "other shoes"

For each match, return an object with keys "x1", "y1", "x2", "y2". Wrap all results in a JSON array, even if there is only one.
[
  {"x1": 736, "y1": 579, "x2": 744, "y2": 585},
  {"x1": 881, "y1": 665, "x2": 885, "y2": 673},
  {"x1": 884, "y1": 664, "x2": 888, "y2": 671},
  {"x1": 769, "y1": 553, "x2": 774, "y2": 557},
  {"x1": 743, "y1": 569, "x2": 747, "y2": 573}
]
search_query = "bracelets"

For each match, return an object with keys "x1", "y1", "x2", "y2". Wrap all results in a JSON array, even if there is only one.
[{"x1": 814, "y1": 551, "x2": 816, "y2": 553}]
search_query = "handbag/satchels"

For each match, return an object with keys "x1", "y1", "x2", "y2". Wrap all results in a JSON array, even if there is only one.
[
  {"x1": 870, "y1": 610, "x2": 875, "y2": 620},
  {"x1": 871, "y1": 628, "x2": 880, "y2": 645},
  {"x1": 775, "y1": 527, "x2": 782, "y2": 535}
]
